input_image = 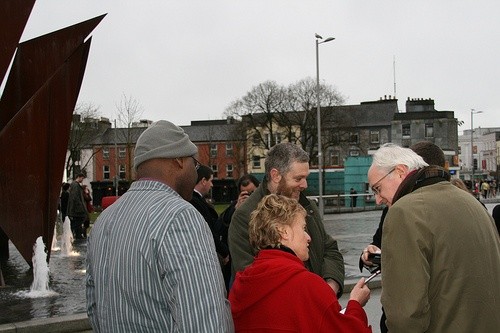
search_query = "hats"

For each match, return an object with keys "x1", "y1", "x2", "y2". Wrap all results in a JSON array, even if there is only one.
[{"x1": 134, "y1": 120, "x2": 198, "y2": 171}]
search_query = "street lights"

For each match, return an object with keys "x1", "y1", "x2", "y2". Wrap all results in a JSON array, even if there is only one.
[
  {"x1": 470, "y1": 108, "x2": 483, "y2": 181},
  {"x1": 313, "y1": 32, "x2": 336, "y2": 222}
]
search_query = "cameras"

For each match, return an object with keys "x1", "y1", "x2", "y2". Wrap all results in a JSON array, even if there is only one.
[
  {"x1": 368, "y1": 253, "x2": 381, "y2": 265},
  {"x1": 243, "y1": 192, "x2": 252, "y2": 196}
]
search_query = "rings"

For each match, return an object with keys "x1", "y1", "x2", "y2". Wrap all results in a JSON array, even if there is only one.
[{"x1": 362, "y1": 249, "x2": 368, "y2": 253}]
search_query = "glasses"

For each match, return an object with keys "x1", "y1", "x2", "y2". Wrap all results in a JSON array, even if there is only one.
[
  {"x1": 191, "y1": 156, "x2": 201, "y2": 171},
  {"x1": 371, "y1": 164, "x2": 408, "y2": 195}
]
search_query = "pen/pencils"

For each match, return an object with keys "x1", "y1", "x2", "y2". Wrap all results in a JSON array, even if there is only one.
[{"x1": 364, "y1": 270, "x2": 381, "y2": 283}]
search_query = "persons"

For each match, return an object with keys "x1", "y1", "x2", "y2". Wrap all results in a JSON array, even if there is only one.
[
  {"x1": 227, "y1": 142, "x2": 345, "y2": 299},
  {"x1": 366, "y1": 144, "x2": 500, "y2": 333},
  {"x1": 84, "y1": 120, "x2": 234, "y2": 333},
  {"x1": 359, "y1": 142, "x2": 450, "y2": 333},
  {"x1": 59, "y1": 165, "x2": 500, "y2": 245},
  {"x1": 229, "y1": 194, "x2": 373, "y2": 333}
]
